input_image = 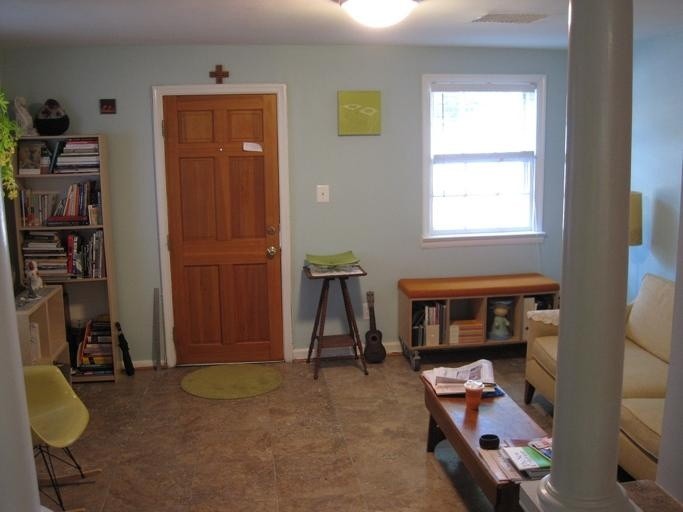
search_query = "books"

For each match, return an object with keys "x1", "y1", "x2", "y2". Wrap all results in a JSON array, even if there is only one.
[
  {"x1": 412, "y1": 300, "x2": 446, "y2": 347},
  {"x1": 18, "y1": 138, "x2": 115, "y2": 377},
  {"x1": 479, "y1": 437, "x2": 553, "y2": 485}
]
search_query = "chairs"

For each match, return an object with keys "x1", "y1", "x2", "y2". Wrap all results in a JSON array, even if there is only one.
[{"x1": 23, "y1": 364, "x2": 102, "y2": 512}]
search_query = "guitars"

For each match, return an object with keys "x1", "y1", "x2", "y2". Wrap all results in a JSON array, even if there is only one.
[{"x1": 364, "y1": 291, "x2": 386, "y2": 364}]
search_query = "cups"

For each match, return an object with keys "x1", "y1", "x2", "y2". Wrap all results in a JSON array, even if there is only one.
[
  {"x1": 464, "y1": 381, "x2": 485, "y2": 410},
  {"x1": 463, "y1": 411, "x2": 479, "y2": 436}
]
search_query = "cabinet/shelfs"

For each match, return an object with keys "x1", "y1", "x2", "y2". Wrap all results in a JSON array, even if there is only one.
[
  {"x1": 398, "y1": 273, "x2": 561, "y2": 371},
  {"x1": 14, "y1": 135, "x2": 121, "y2": 384}
]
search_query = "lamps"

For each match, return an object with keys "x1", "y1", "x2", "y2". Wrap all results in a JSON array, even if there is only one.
[{"x1": 628, "y1": 192, "x2": 644, "y2": 246}]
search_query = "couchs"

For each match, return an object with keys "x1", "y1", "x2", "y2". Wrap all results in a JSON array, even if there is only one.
[{"x1": 523, "y1": 273, "x2": 675, "y2": 482}]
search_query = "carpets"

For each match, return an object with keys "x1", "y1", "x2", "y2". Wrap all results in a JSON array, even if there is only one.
[{"x1": 180, "y1": 363, "x2": 282, "y2": 400}]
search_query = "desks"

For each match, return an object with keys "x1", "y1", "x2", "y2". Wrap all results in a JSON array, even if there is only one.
[{"x1": 303, "y1": 264, "x2": 368, "y2": 380}]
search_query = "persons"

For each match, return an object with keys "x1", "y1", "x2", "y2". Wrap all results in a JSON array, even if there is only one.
[{"x1": 488, "y1": 299, "x2": 513, "y2": 340}]
